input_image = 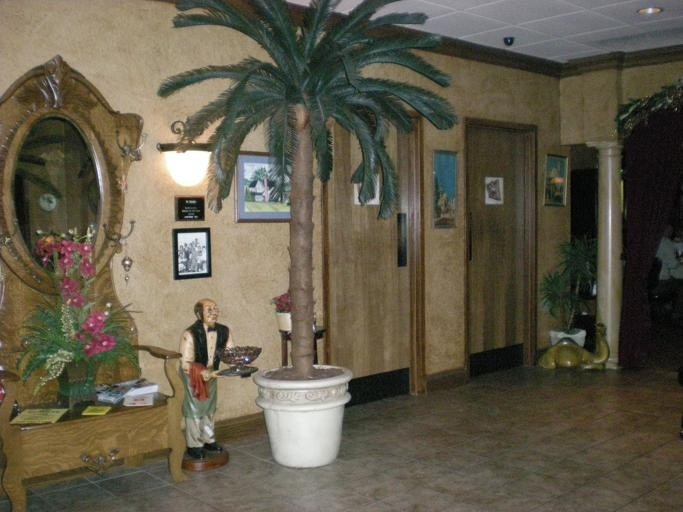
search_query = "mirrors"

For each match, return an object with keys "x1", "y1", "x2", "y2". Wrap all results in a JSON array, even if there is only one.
[{"x1": 2, "y1": 53, "x2": 144, "y2": 295}]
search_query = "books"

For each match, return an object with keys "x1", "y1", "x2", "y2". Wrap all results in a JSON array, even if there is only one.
[{"x1": 97, "y1": 378, "x2": 159, "y2": 407}]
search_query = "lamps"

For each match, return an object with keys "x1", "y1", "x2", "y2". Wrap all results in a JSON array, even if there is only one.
[{"x1": 156, "y1": 117, "x2": 214, "y2": 187}]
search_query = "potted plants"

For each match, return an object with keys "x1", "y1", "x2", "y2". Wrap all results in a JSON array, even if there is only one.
[{"x1": 540, "y1": 232, "x2": 594, "y2": 347}]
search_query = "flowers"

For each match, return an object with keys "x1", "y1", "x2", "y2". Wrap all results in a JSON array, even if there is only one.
[
  {"x1": 13, "y1": 229, "x2": 154, "y2": 391},
  {"x1": 268, "y1": 293, "x2": 291, "y2": 313}
]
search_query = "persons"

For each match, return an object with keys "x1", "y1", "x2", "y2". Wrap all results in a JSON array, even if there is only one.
[
  {"x1": 649, "y1": 224, "x2": 683, "y2": 338},
  {"x1": 178, "y1": 298, "x2": 234, "y2": 459},
  {"x1": 177, "y1": 238, "x2": 206, "y2": 273}
]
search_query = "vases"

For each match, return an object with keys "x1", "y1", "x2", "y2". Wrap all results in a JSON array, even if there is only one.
[
  {"x1": 274, "y1": 310, "x2": 291, "y2": 331},
  {"x1": 548, "y1": 328, "x2": 587, "y2": 347},
  {"x1": 54, "y1": 361, "x2": 102, "y2": 414}
]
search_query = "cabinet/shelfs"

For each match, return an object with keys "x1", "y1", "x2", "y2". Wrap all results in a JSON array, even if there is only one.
[{"x1": 279, "y1": 327, "x2": 325, "y2": 366}]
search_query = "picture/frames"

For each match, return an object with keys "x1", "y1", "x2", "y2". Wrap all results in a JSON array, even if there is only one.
[
  {"x1": 428, "y1": 150, "x2": 460, "y2": 228},
  {"x1": 171, "y1": 227, "x2": 212, "y2": 279},
  {"x1": 543, "y1": 153, "x2": 574, "y2": 211},
  {"x1": 228, "y1": 151, "x2": 294, "y2": 222}
]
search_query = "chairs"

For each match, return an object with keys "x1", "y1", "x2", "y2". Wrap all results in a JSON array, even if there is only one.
[
  {"x1": 1, "y1": 342, "x2": 192, "y2": 512},
  {"x1": 156, "y1": 1, "x2": 462, "y2": 474}
]
